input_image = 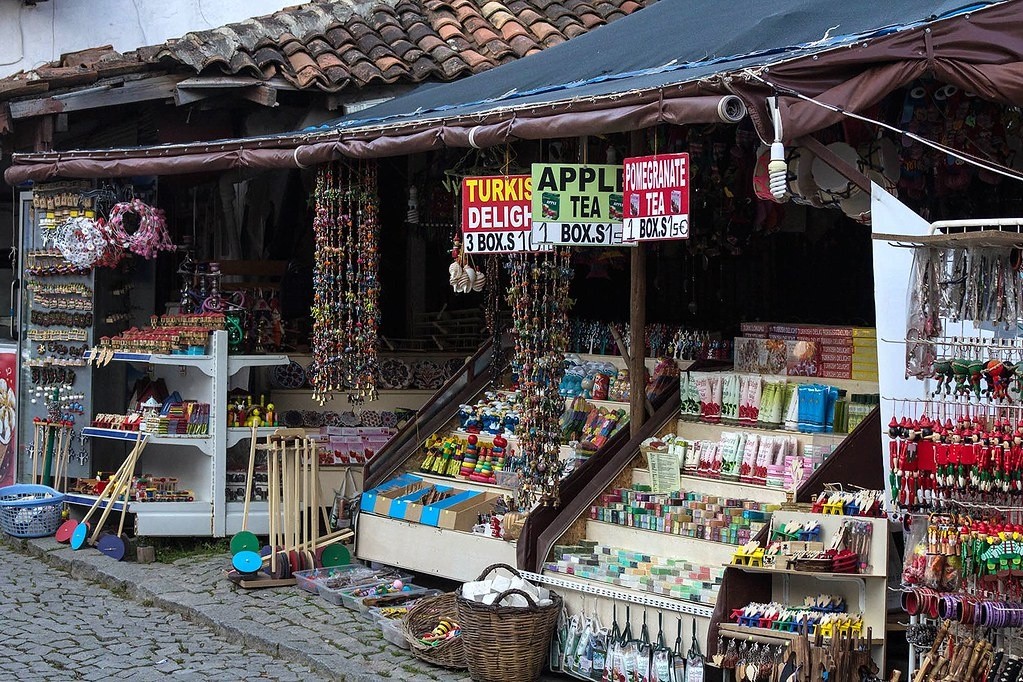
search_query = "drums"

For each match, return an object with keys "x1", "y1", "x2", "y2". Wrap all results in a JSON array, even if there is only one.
[{"x1": 753, "y1": 127, "x2": 899, "y2": 228}]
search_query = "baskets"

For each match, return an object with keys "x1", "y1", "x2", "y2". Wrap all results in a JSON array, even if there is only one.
[
  {"x1": 400, "y1": 591, "x2": 468, "y2": 668},
  {"x1": 1, "y1": 483, "x2": 65, "y2": 538},
  {"x1": 455, "y1": 563, "x2": 563, "y2": 680}
]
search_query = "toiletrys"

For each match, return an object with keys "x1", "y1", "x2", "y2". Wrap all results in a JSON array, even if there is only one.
[
  {"x1": 668, "y1": 431, "x2": 797, "y2": 486},
  {"x1": 680, "y1": 371, "x2": 787, "y2": 434},
  {"x1": 779, "y1": 383, "x2": 880, "y2": 434}
]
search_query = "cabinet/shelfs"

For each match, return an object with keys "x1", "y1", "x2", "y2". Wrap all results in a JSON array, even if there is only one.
[
  {"x1": 254, "y1": 353, "x2": 695, "y2": 507},
  {"x1": 354, "y1": 352, "x2": 734, "y2": 593},
  {"x1": 64, "y1": 330, "x2": 304, "y2": 550},
  {"x1": 537, "y1": 370, "x2": 902, "y2": 682},
  {"x1": 717, "y1": 511, "x2": 888, "y2": 682}
]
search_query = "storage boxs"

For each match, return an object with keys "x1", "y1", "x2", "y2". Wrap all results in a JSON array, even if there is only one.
[
  {"x1": 494, "y1": 470, "x2": 519, "y2": 488},
  {"x1": 254, "y1": 486, "x2": 283, "y2": 500},
  {"x1": 360, "y1": 475, "x2": 504, "y2": 532},
  {"x1": 293, "y1": 564, "x2": 445, "y2": 649},
  {"x1": 462, "y1": 575, "x2": 553, "y2": 606},
  {"x1": 301, "y1": 426, "x2": 398, "y2": 465},
  {"x1": 742, "y1": 322, "x2": 879, "y2": 381},
  {"x1": 225, "y1": 486, "x2": 247, "y2": 502},
  {"x1": 254, "y1": 470, "x2": 283, "y2": 485},
  {"x1": 227, "y1": 470, "x2": 248, "y2": 485}
]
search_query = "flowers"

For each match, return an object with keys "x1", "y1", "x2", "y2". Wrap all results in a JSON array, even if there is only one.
[{"x1": 54, "y1": 199, "x2": 178, "y2": 272}]
